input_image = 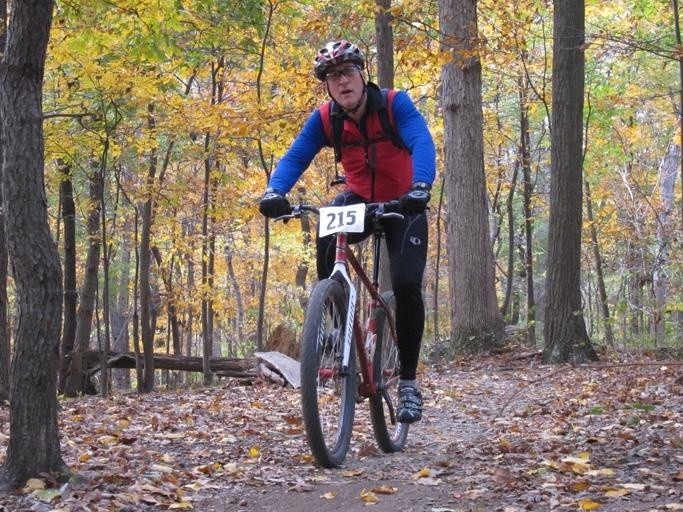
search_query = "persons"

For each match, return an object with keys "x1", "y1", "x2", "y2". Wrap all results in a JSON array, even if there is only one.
[{"x1": 257, "y1": 39, "x2": 437, "y2": 425}]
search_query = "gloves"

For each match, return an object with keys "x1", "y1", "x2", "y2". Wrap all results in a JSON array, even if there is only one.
[
  {"x1": 400, "y1": 182, "x2": 431, "y2": 216},
  {"x1": 260, "y1": 189, "x2": 292, "y2": 223}
]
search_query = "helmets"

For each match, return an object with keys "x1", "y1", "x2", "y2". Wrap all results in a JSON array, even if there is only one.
[{"x1": 314, "y1": 40, "x2": 364, "y2": 81}]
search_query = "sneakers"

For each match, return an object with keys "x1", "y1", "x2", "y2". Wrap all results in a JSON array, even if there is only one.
[
  {"x1": 396, "y1": 386, "x2": 422, "y2": 422},
  {"x1": 320, "y1": 331, "x2": 340, "y2": 354}
]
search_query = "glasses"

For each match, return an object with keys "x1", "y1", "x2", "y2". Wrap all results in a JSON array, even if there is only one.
[{"x1": 323, "y1": 66, "x2": 358, "y2": 81}]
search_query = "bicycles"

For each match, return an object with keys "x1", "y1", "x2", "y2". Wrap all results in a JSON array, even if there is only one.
[{"x1": 271, "y1": 197, "x2": 411, "y2": 467}]
